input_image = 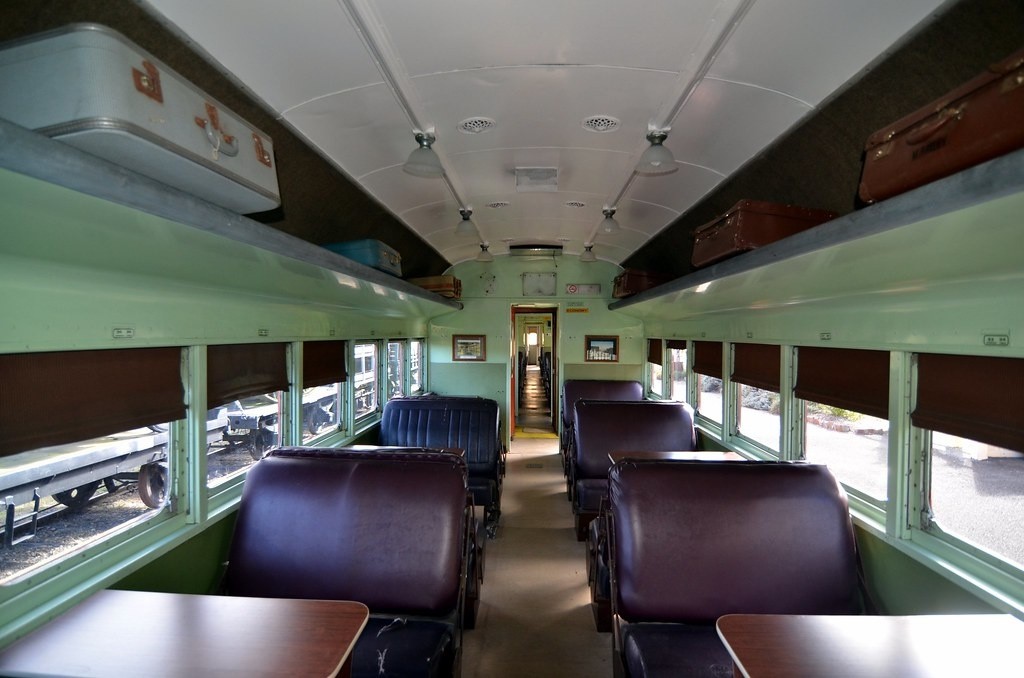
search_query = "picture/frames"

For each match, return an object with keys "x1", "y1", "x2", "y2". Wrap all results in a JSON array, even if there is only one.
[
  {"x1": 452, "y1": 335, "x2": 486, "y2": 361},
  {"x1": 584, "y1": 335, "x2": 619, "y2": 362}
]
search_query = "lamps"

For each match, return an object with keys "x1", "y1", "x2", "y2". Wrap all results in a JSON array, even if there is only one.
[
  {"x1": 634, "y1": 130, "x2": 679, "y2": 174},
  {"x1": 597, "y1": 209, "x2": 623, "y2": 235},
  {"x1": 402, "y1": 132, "x2": 445, "y2": 179},
  {"x1": 579, "y1": 245, "x2": 598, "y2": 262},
  {"x1": 476, "y1": 244, "x2": 494, "y2": 262},
  {"x1": 454, "y1": 210, "x2": 478, "y2": 233}
]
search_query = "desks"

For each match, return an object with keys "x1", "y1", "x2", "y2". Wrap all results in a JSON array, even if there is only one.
[
  {"x1": 339, "y1": 444, "x2": 466, "y2": 457},
  {"x1": 0, "y1": 589, "x2": 370, "y2": 678},
  {"x1": 715, "y1": 613, "x2": 1023, "y2": 678},
  {"x1": 607, "y1": 450, "x2": 748, "y2": 461},
  {"x1": 417, "y1": 395, "x2": 483, "y2": 400}
]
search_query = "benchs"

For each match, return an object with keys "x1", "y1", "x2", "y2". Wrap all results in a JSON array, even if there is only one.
[
  {"x1": 571, "y1": 403, "x2": 700, "y2": 542},
  {"x1": 561, "y1": 400, "x2": 688, "y2": 502},
  {"x1": 214, "y1": 457, "x2": 467, "y2": 678},
  {"x1": 379, "y1": 399, "x2": 506, "y2": 540},
  {"x1": 585, "y1": 459, "x2": 811, "y2": 633},
  {"x1": 604, "y1": 464, "x2": 881, "y2": 678},
  {"x1": 262, "y1": 445, "x2": 488, "y2": 631},
  {"x1": 560, "y1": 379, "x2": 643, "y2": 451}
]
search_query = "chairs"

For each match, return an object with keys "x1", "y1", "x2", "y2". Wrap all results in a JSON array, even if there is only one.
[{"x1": 517, "y1": 350, "x2": 552, "y2": 410}]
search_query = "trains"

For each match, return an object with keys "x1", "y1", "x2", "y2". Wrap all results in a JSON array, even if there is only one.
[{"x1": 0, "y1": 343, "x2": 421, "y2": 549}]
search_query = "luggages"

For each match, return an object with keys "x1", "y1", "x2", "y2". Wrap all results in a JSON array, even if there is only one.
[
  {"x1": 0, "y1": 20, "x2": 282, "y2": 222},
  {"x1": 853, "y1": 47, "x2": 1024, "y2": 210},
  {"x1": 611, "y1": 265, "x2": 668, "y2": 299},
  {"x1": 690, "y1": 197, "x2": 841, "y2": 271},
  {"x1": 405, "y1": 274, "x2": 462, "y2": 299},
  {"x1": 321, "y1": 237, "x2": 403, "y2": 278}
]
